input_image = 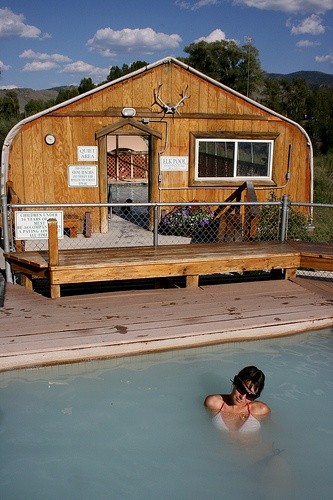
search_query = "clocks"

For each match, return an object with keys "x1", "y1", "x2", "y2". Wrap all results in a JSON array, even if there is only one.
[{"x1": 45, "y1": 135, "x2": 56, "y2": 145}]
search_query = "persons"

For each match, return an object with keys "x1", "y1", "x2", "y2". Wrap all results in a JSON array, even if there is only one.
[
  {"x1": 120, "y1": 199, "x2": 133, "y2": 215},
  {"x1": 204, "y1": 366, "x2": 270, "y2": 414}
]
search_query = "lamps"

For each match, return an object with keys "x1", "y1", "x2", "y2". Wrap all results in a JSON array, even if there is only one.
[{"x1": 122, "y1": 107, "x2": 136, "y2": 116}]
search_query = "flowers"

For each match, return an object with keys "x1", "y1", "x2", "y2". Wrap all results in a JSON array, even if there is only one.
[{"x1": 162, "y1": 199, "x2": 220, "y2": 229}]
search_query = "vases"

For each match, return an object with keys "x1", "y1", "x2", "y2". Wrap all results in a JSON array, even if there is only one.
[{"x1": 159, "y1": 223, "x2": 218, "y2": 243}]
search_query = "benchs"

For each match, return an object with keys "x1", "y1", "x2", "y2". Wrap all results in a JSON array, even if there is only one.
[{"x1": 63, "y1": 214, "x2": 79, "y2": 238}]
